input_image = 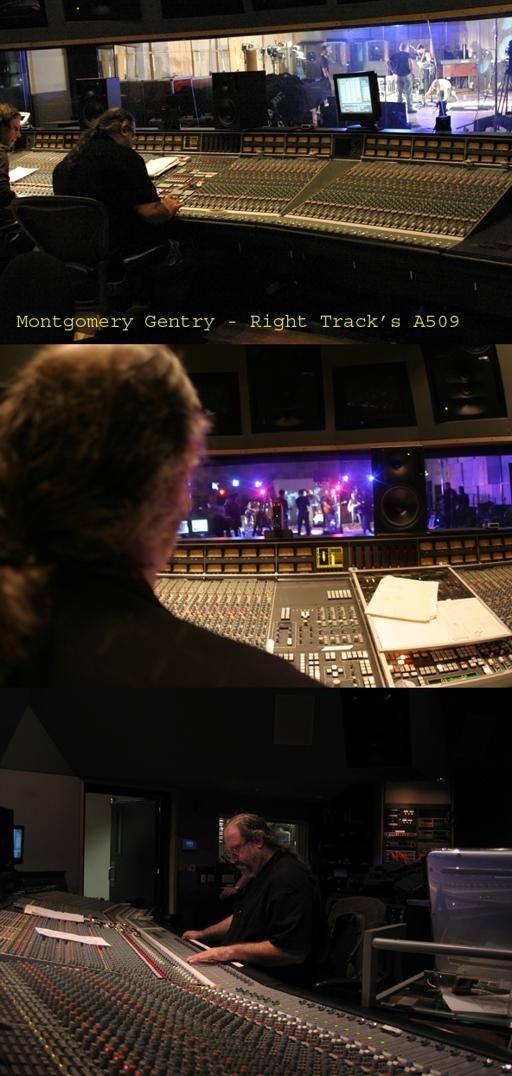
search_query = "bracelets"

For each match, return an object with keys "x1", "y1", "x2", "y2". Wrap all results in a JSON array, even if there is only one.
[{"x1": 233, "y1": 884, "x2": 241, "y2": 892}]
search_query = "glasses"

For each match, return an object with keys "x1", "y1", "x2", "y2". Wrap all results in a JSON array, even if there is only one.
[{"x1": 223, "y1": 838, "x2": 254, "y2": 860}]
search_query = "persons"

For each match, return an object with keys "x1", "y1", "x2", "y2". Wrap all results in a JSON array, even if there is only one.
[
  {"x1": 422, "y1": 78, "x2": 459, "y2": 103},
  {"x1": 444, "y1": 44, "x2": 468, "y2": 88},
  {"x1": 182, "y1": 807, "x2": 312, "y2": 965},
  {"x1": 318, "y1": 43, "x2": 336, "y2": 88},
  {"x1": 416, "y1": 43, "x2": 432, "y2": 96},
  {"x1": 444, "y1": 482, "x2": 457, "y2": 527},
  {"x1": 223, "y1": 483, "x2": 373, "y2": 537},
  {"x1": 51, "y1": 106, "x2": 186, "y2": 257},
  {"x1": 386, "y1": 41, "x2": 418, "y2": 113},
  {"x1": 216, "y1": 875, "x2": 250, "y2": 900},
  {"x1": 1, "y1": 343, "x2": 349, "y2": 773},
  {"x1": 456, "y1": 485, "x2": 470, "y2": 512},
  {"x1": 0, "y1": 100, "x2": 21, "y2": 207}
]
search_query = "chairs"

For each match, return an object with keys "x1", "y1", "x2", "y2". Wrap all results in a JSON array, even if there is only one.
[
  {"x1": 314, "y1": 897, "x2": 391, "y2": 1008},
  {"x1": 14, "y1": 195, "x2": 182, "y2": 312}
]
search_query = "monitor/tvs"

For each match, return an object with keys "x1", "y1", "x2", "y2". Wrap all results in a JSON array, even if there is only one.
[
  {"x1": 13, "y1": 826, "x2": 25, "y2": 864},
  {"x1": 332, "y1": 71, "x2": 388, "y2": 131}
]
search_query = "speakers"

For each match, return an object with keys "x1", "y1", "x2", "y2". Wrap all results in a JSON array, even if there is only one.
[
  {"x1": 373, "y1": 445, "x2": 427, "y2": 536},
  {"x1": 212, "y1": 70, "x2": 267, "y2": 130},
  {"x1": 73, "y1": 77, "x2": 122, "y2": 118}
]
